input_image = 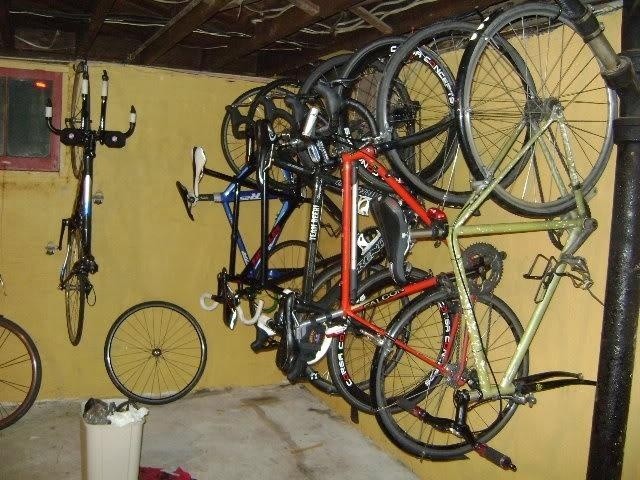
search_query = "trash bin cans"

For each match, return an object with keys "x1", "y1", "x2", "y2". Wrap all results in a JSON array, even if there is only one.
[{"x1": 79, "y1": 398, "x2": 146, "y2": 480}]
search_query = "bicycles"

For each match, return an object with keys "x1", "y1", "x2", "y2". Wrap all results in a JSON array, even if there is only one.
[
  {"x1": 189, "y1": 75, "x2": 333, "y2": 326},
  {"x1": 274, "y1": 19, "x2": 539, "y2": 462},
  {"x1": 43, "y1": 61, "x2": 137, "y2": 347},
  {"x1": 369, "y1": 0, "x2": 620, "y2": 473},
  {"x1": 254, "y1": 35, "x2": 460, "y2": 415},
  {"x1": 223, "y1": 50, "x2": 416, "y2": 396},
  {"x1": 175, "y1": 86, "x2": 299, "y2": 354}
]
